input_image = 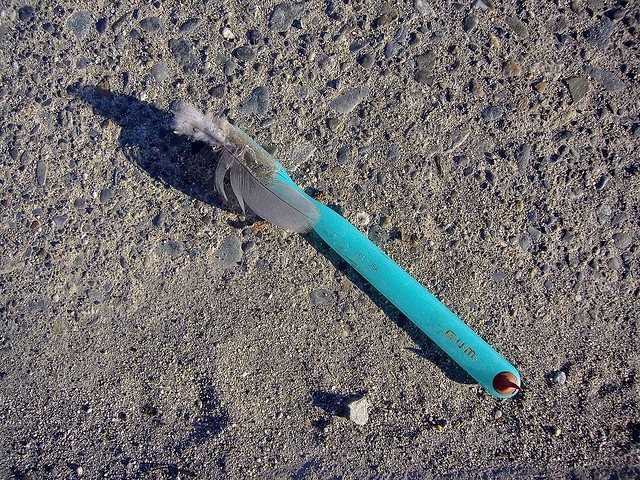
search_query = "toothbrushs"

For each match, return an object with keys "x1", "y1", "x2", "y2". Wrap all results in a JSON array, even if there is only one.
[{"x1": 205, "y1": 118, "x2": 522, "y2": 400}]
